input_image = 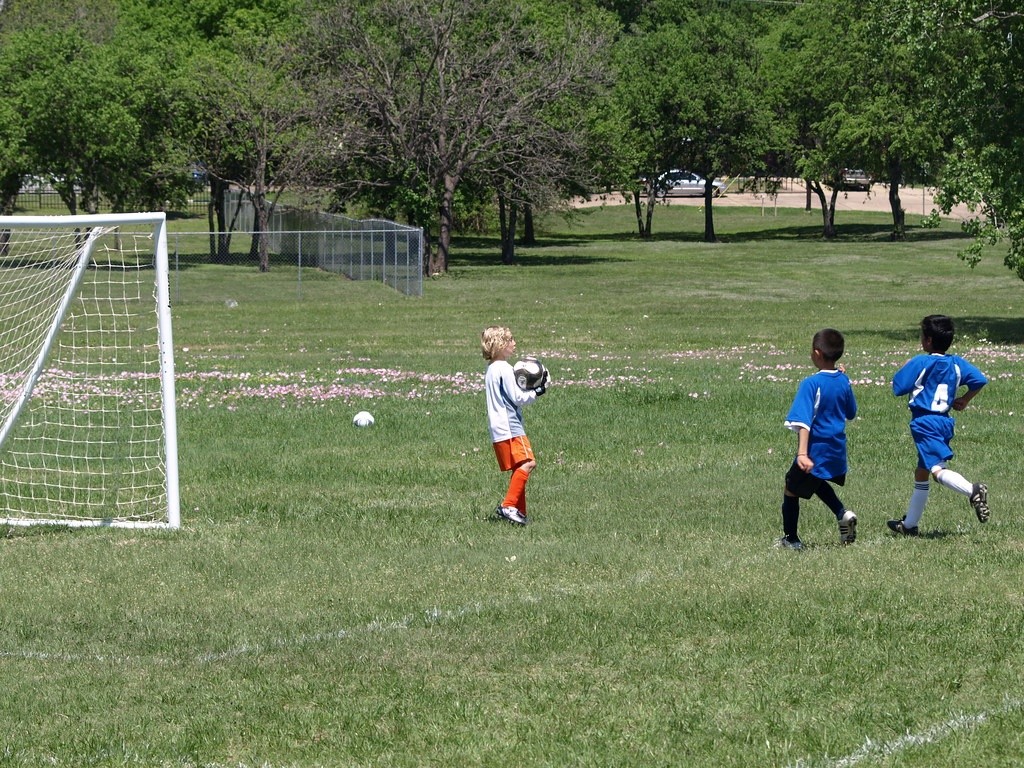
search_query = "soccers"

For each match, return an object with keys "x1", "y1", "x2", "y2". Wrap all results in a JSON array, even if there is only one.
[
  {"x1": 513, "y1": 356, "x2": 544, "y2": 391},
  {"x1": 353, "y1": 411, "x2": 375, "y2": 427}
]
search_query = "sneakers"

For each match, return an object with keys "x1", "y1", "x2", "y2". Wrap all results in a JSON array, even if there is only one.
[
  {"x1": 837, "y1": 510, "x2": 858, "y2": 545},
  {"x1": 887, "y1": 515, "x2": 918, "y2": 537},
  {"x1": 970, "y1": 482, "x2": 990, "y2": 523},
  {"x1": 496, "y1": 503, "x2": 528, "y2": 525},
  {"x1": 773, "y1": 535, "x2": 801, "y2": 549}
]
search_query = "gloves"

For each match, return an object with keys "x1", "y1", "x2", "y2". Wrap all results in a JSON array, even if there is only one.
[{"x1": 534, "y1": 386, "x2": 546, "y2": 396}]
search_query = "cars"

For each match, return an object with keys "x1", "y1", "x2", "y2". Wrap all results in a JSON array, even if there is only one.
[
  {"x1": 649, "y1": 168, "x2": 728, "y2": 198},
  {"x1": 821, "y1": 167, "x2": 873, "y2": 191}
]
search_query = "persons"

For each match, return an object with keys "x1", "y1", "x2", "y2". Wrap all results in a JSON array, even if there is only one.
[
  {"x1": 775, "y1": 328, "x2": 858, "y2": 549},
  {"x1": 887, "y1": 315, "x2": 990, "y2": 536},
  {"x1": 481, "y1": 325, "x2": 551, "y2": 528}
]
